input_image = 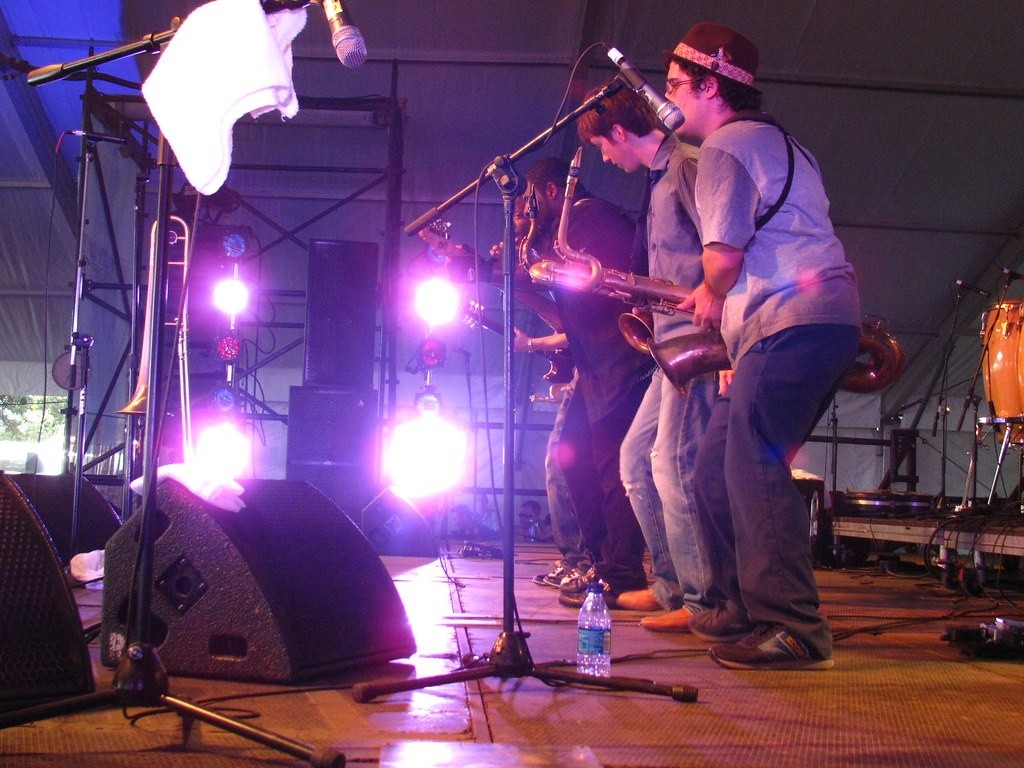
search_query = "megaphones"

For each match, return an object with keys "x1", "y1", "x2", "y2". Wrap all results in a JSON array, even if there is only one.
[{"x1": 320, "y1": 0, "x2": 367, "y2": 68}]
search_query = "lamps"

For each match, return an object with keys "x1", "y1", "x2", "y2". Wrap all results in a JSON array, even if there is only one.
[
  {"x1": 413, "y1": 245, "x2": 447, "y2": 417},
  {"x1": 209, "y1": 233, "x2": 250, "y2": 414}
]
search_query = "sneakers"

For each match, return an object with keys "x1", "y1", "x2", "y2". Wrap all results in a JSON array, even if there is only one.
[
  {"x1": 533, "y1": 563, "x2": 582, "y2": 585},
  {"x1": 689, "y1": 605, "x2": 762, "y2": 642},
  {"x1": 709, "y1": 607, "x2": 836, "y2": 671}
]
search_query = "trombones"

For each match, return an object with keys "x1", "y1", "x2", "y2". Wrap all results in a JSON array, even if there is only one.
[{"x1": 113, "y1": 212, "x2": 192, "y2": 480}]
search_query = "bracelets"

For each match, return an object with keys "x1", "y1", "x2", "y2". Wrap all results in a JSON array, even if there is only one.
[{"x1": 527, "y1": 337, "x2": 533, "y2": 355}]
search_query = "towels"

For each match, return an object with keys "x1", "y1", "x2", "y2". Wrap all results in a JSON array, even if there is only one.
[
  {"x1": 141, "y1": 0, "x2": 308, "y2": 198},
  {"x1": 70, "y1": 549, "x2": 105, "y2": 582},
  {"x1": 128, "y1": 464, "x2": 248, "y2": 515}
]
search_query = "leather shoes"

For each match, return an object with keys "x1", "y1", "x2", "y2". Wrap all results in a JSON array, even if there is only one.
[
  {"x1": 617, "y1": 586, "x2": 668, "y2": 614},
  {"x1": 641, "y1": 605, "x2": 703, "y2": 631},
  {"x1": 562, "y1": 587, "x2": 617, "y2": 608},
  {"x1": 559, "y1": 570, "x2": 600, "y2": 591}
]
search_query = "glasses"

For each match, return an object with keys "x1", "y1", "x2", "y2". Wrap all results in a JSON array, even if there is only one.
[
  {"x1": 665, "y1": 75, "x2": 710, "y2": 93},
  {"x1": 518, "y1": 512, "x2": 534, "y2": 519}
]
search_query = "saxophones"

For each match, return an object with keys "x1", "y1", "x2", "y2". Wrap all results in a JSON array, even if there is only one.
[
  {"x1": 526, "y1": 144, "x2": 908, "y2": 395},
  {"x1": 617, "y1": 309, "x2": 655, "y2": 354},
  {"x1": 515, "y1": 181, "x2": 535, "y2": 274}
]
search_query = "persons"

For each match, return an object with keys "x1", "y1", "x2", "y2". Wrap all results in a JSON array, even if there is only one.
[
  {"x1": 488, "y1": 83, "x2": 715, "y2": 633},
  {"x1": 677, "y1": 21, "x2": 865, "y2": 669}
]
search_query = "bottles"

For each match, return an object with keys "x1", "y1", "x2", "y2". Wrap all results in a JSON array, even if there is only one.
[
  {"x1": 526, "y1": 518, "x2": 538, "y2": 542},
  {"x1": 578, "y1": 584, "x2": 612, "y2": 680}
]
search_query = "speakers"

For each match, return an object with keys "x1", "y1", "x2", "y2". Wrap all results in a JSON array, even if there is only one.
[
  {"x1": 0, "y1": 0, "x2": 346, "y2": 768},
  {"x1": 361, "y1": 485, "x2": 440, "y2": 557},
  {"x1": 285, "y1": 385, "x2": 379, "y2": 531},
  {"x1": 0, "y1": 471, "x2": 97, "y2": 714},
  {"x1": 302, "y1": 239, "x2": 379, "y2": 387},
  {"x1": 100, "y1": 477, "x2": 417, "y2": 681}
]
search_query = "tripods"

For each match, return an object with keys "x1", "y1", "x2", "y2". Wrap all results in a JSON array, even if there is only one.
[{"x1": 351, "y1": 79, "x2": 700, "y2": 705}]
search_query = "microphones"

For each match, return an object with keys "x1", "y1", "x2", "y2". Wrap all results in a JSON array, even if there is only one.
[
  {"x1": 1002, "y1": 268, "x2": 1024, "y2": 280},
  {"x1": 955, "y1": 279, "x2": 991, "y2": 297},
  {"x1": 65, "y1": 129, "x2": 128, "y2": 145},
  {"x1": 601, "y1": 43, "x2": 685, "y2": 131}
]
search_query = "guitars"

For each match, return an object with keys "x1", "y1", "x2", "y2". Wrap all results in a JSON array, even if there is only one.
[
  {"x1": 419, "y1": 216, "x2": 564, "y2": 334},
  {"x1": 451, "y1": 297, "x2": 583, "y2": 384}
]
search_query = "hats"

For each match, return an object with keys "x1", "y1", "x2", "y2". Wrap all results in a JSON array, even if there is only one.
[{"x1": 663, "y1": 22, "x2": 761, "y2": 94}]
